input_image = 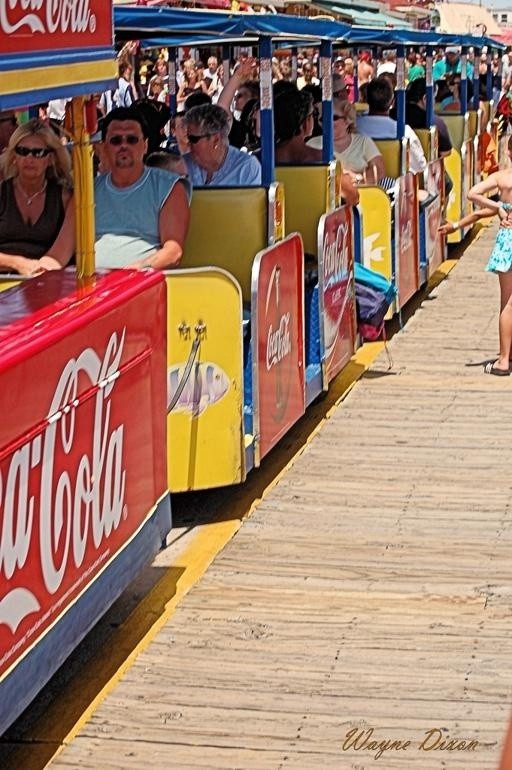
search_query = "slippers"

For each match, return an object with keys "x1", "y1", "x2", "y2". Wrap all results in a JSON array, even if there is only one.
[{"x1": 484, "y1": 359, "x2": 511, "y2": 375}]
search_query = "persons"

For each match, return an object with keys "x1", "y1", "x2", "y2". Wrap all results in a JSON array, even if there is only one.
[
  {"x1": 467, "y1": 137, "x2": 512, "y2": 377},
  {"x1": 0, "y1": 47, "x2": 512, "y2": 275}
]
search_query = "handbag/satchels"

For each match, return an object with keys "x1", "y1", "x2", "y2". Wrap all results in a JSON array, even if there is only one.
[{"x1": 354, "y1": 261, "x2": 397, "y2": 328}]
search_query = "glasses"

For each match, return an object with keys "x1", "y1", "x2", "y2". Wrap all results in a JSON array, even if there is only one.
[
  {"x1": 187, "y1": 134, "x2": 206, "y2": 144},
  {"x1": 319, "y1": 114, "x2": 346, "y2": 123},
  {"x1": 109, "y1": 135, "x2": 139, "y2": 145},
  {"x1": 15, "y1": 145, "x2": 51, "y2": 158}
]
